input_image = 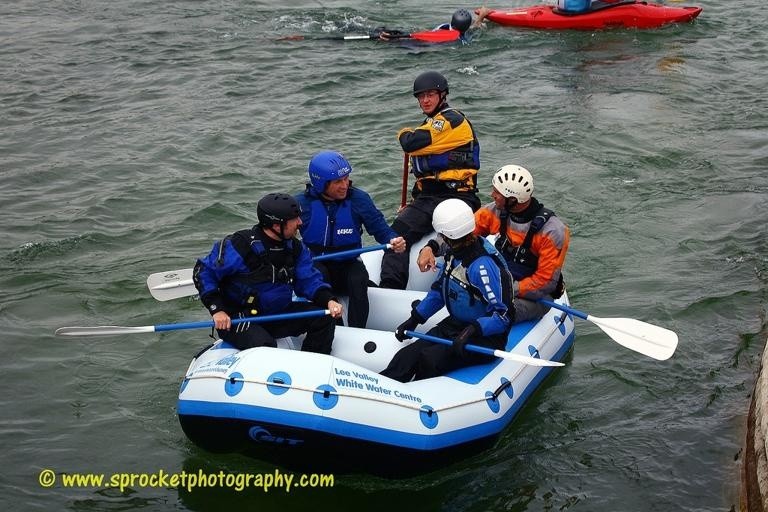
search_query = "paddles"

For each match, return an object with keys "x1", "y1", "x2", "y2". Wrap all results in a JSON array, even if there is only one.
[
  {"x1": 55, "y1": 306, "x2": 338, "y2": 335},
  {"x1": 370, "y1": 30, "x2": 461, "y2": 42},
  {"x1": 146, "y1": 237, "x2": 398, "y2": 301},
  {"x1": 396, "y1": 329, "x2": 566, "y2": 367},
  {"x1": 427, "y1": 261, "x2": 678, "y2": 360}
]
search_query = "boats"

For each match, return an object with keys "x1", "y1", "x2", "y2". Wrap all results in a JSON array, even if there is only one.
[
  {"x1": 475, "y1": 4, "x2": 702, "y2": 28},
  {"x1": 176, "y1": 233, "x2": 575, "y2": 463}
]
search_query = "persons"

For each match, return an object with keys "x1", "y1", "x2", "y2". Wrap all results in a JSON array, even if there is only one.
[
  {"x1": 293, "y1": 151, "x2": 407, "y2": 328},
  {"x1": 553, "y1": 0, "x2": 608, "y2": 16},
  {"x1": 417, "y1": 165, "x2": 569, "y2": 323},
  {"x1": 331, "y1": 7, "x2": 495, "y2": 49},
  {"x1": 377, "y1": 197, "x2": 516, "y2": 382},
  {"x1": 369, "y1": 71, "x2": 481, "y2": 290},
  {"x1": 192, "y1": 191, "x2": 345, "y2": 355}
]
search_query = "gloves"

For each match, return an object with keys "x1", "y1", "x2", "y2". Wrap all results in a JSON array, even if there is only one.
[
  {"x1": 451, "y1": 319, "x2": 483, "y2": 353},
  {"x1": 395, "y1": 306, "x2": 426, "y2": 342}
]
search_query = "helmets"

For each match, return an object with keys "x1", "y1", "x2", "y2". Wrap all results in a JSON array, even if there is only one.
[
  {"x1": 413, "y1": 71, "x2": 449, "y2": 98},
  {"x1": 309, "y1": 151, "x2": 352, "y2": 194},
  {"x1": 452, "y1": 9, "x2": 472, "y2": 33},
  {"x1": 491, "y1": 165, "x2": 535, "y2": 204},
  {"x1": 432, "y1": 198, "x2": 476, "y2": 240},
  {"x1": 257, "y1": 193, "x2": 303, "y2": 229}
]
